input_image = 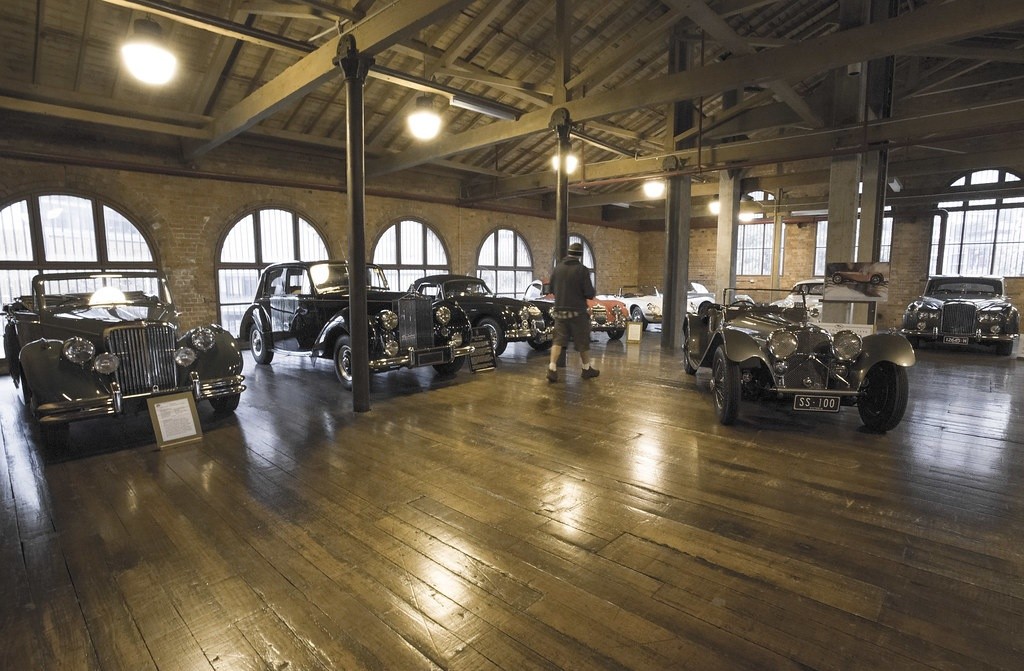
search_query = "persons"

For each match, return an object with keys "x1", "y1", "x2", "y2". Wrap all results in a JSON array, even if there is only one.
[{"x1": 546, "y1": 242, "x2": 600, "y2": 382}]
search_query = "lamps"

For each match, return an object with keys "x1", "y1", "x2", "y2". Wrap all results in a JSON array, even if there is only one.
[
  {"x1": 120, "y1": 12, "x2": 177, "y2": 85},
  {"x1": 552, "y1": 142, "x2": 577, "y2": 175},
  {"x1": 407, "y1": 29, "x2": 442, "y2": 141}
]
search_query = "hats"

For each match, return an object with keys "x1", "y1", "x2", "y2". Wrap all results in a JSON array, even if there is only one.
[{"x1": 567, "y1": 243, "x2": 582, "y2": 252}]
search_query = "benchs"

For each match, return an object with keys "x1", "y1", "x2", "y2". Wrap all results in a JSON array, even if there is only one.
[
  {"x1": 15, "y1": 291, "x2": 144, "y2": 311},
  {"x1": 707, "y1": 308, "x2": 802, "y2": 342}
]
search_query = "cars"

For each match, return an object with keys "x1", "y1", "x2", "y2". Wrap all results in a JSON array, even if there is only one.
[
  {"x1": 596, "y1": 282, "x2": 715, "y2": 331},
  {"x1": 831, "y1": 270, "x2": 884, "y2": 284},
  {"x1": 901, "y1": 275, "x2": 1020, "y2": 355},
  {"x1": 239, "y1": 260, "x2": 476, "y2": 391},
  {"x1": 682, "y1": 287, "x2": 915, "y2": 431},
  {"x1": 769, "y1": 278, "x2": 825, "y2": 322},
  {"x1": 406, "y1": 275, "x2": 556, "y2": 357},
  {"x1": 523, "y1": 279, "x2": 630, "y2": 340},
  {"x1": 0, "y1": 271, "x2": 247, "y2": 448}
]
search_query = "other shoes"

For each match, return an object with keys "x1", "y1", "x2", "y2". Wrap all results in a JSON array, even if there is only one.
[
  {"x1": 581, "y1": 366, "x2": 599, "y2": 378},
  {"x1": 546, "y1": 369, "x2": 557, "y2": 382}
]
